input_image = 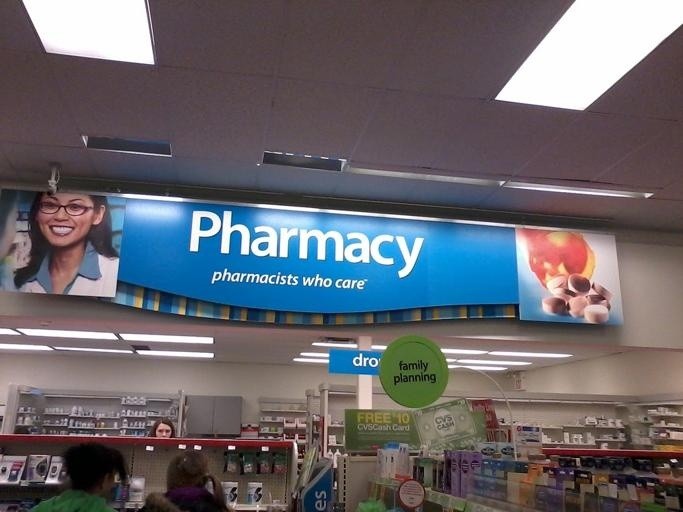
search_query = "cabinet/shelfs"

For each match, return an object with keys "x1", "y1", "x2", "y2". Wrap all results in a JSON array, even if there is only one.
[
  {"x1": 257, "y1": 383, "x2": 683, "y2": 511},
  {"x1": 0, "y1": 382, "x2": 185, "y2": 438}
]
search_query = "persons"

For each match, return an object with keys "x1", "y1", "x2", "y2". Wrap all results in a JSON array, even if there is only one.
[
  {"x1": 27, "y1": 442, "x2": 121, "y2": 512},
  {"x1": 0, "y1": 192, "x2": 119, "y2": 297},
  {"x1": 148, "y1": 417, "x2": 176, "y2": 437},
  {"x1": 141, "y1": 451, "x2": 233, "y2": 512}
]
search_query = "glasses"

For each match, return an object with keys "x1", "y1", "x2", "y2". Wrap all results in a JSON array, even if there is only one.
[{"x1": 37, "y1": 201, "x2": 95, "y2": 217}]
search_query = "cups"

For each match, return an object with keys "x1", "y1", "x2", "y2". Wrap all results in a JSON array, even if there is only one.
[{"x1": 220, "y1": 481, "x2": 264, "y2": 509}]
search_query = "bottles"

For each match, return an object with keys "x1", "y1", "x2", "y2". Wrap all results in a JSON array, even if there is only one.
[
  {"x1": 121, "y1": 408, "x2": 145, "y2": 428},
  {"x1": 55, "y1": 417, "x2": 69, "y2": 427},
  {"x1": 326, "y1": 448, "x2": 341, "y2": 470}
]
